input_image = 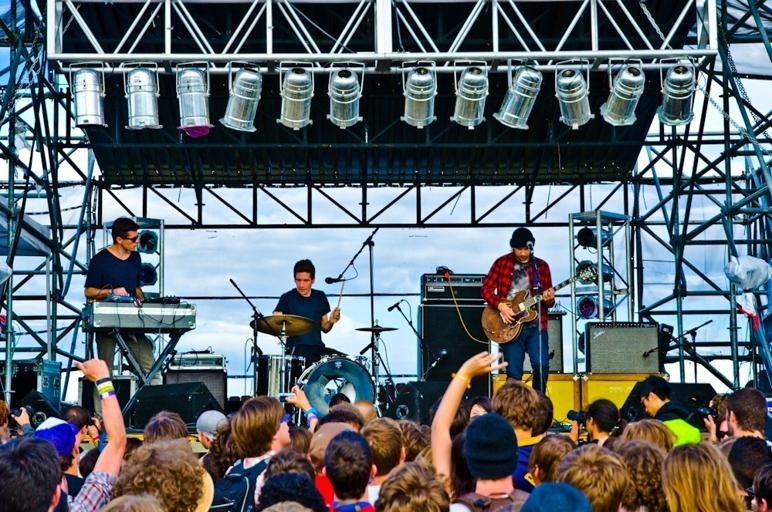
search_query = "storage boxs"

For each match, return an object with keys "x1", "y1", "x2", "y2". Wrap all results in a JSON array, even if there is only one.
[
  {"x1": 582, "y1": 375, "x2": 661, "y2": 411},
  {"x1": 492, "y1": 374, "x2": 580, "y2": 426},
  {"x1": 417, "y1": 304, "x2": 491, "y2": 380}
]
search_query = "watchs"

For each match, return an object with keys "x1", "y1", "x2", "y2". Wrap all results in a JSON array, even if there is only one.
[{"x1": 304, "y1": 408, "x2": 319, "y2": 418}]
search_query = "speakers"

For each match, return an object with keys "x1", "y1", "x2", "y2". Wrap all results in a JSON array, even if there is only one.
[
  {"x1": 619, "y1": 380, "x2": 717, "y2": 424},
  {"x1": 384, "y1": 380, "x2": 450, "y2": 426},
  {"x1": 20, "y1": 389, "x2": 61, "y2": 431},
  {"x1": 133, "y1": 381, "x2": 225, "y2": 430},
  {"x1": 497, "y1": 312, "x2": 565, "y2": 375}
]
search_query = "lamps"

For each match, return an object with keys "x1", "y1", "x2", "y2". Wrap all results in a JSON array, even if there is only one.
[
  {"x1": 600, "y1": 59, "x2": 646, "y2": 126},
  {"x1": 141, "y1": 262, "x2": 157, "y2": 287},
  {"x1": 576, "y1": 259, "x2": 616, "y2": 285},
  {"x1": 449, "y1": 59, "x2": 489, "y2": 131},
  {"x1": 658, "y1": 54, "x2": 697, "y2": 128},
  {"x1": 123, "y1": 61, "x2": 164, "y2": 132},
  {"x1": 136, "y1": 230, "x2": 158, "y2": 255},
  {"x1": 577, "y1": 294, "x2": 616, "y2": 320},
  {"x1": 554, "y1": 58, "x2": 595, "y2": 130},
  {"x1": 218, "y1": 62, "x2": 264, "y2": 134},
  {"x1": 493, "y1": 58, "x2": 543, "y2": 132},
  {"x1": 326, "y1": 59, "x2": 363, "y2": 131},
  {"x1": 174, "y1": 60, "x2": 216, "y2": 139},
  {"x1": 577, "y1": 228, "x2": 610, "y2": 249},
  {"x1": 67, "y1": 61, "x2": 110, "y2": 129},
  {"x1": 276, "y1": 60, "x2": 313, "y2": 132},
  {"x1": 400, "y1": 60, "x2": 437, "y2": 129}
]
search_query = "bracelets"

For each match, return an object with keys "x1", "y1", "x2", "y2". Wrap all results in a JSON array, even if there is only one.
[
  {"x1": 95, "y1": 376, "x2": 116, "y2": 399},
  {"x1": 92, "y1": 434, "x2": 99, "y2": 445},
  {"x1": 306, "y1": 416, "x2": 319, "y2": 426},
  {"x1": 451, "y1": 372, "x2": 471, "y2": 389}
]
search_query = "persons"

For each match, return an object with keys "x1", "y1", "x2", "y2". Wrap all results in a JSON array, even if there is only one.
[
  {"x1": 196, "y1": 409, "x2": 229, "y2": 467},
  {"x1": 353, "y1": 398, "x2": 377, "y2": 426},
  {"x1": 373, "y1": 462, "x2": 450, "y2": 512},
  {"x1": 467, "y1": 398, "x2": 493, "y2": 421},
  {"x1": 616, "y1": 440, "x2": 669, "y2": 512},
  {"x1": 451, "y1": 411, "x2": 527, "y2": 512},
  {"x1": 264, "y1": 450, "x2": 315, "y2": 482},
  {"x1": 223, "y1": 396, "x2": 291, "y2": 511},
  {"x1": 431, "y1": 351, "x2": 508, "y2": 502},
  {"x1": 283, "y1": 429, "x2": 313, "y2": 452},
  {"x1": 532, "y1": 389, "x2": 554, "y2": 442},
  {"x1": 398, "y1": 418, "x2": 432, "y2": 463},
  {"x1": 0, "y1": 436, "x2": 62, "y2": 512},
  {"x1": 210, "y1": 424, "x2": 241, "y2": 479},
  {"x1": 108, "y1": 438, "x2": 204, "y2": 512},
  {"x1": 570, "y1": 399, "x2": 628, "y2": 451},
  {"x1": 660, "y1": 442, "x2": 751, "y2": 512},
  {"x1": 285, "y1": 384, "x2": 318, "y2": 460},
  {"x1": 143, "y1": 411, "x2": 187, "y2": 444},
  {"x1": 330, "y1": 403, "x2": 365, "y2": 425},
  {"x1": 751, "y1": 464, "x2": 772, "y2": 512},
  {"x1": 528, "y1": 434, "x2": 577, "y2": 487},
  {"x1": 322, "y1": 430, "x2": 378, "y2": 512},
  {"x1": 34, "y1": 358, "x2": 127, "y2": 512},
  {"x1": 360, "y1": 417, "x2": 407, "y2": 507},
  {"x1": 704, "y1": 387, "x2": 772, "y2": 447},
  {"x1": 314, "y1": 410, "x2": 363, "y2": 432},
  {"x1": 59, "y1": 405, "x2": 101, "y2": 477},
  {"x1": 706, "y1": 394, "x2": 730, "y2": 438},
  {"x1": 307, "y1": 422, "x2": 359, "y2": 508},
  {"x1": 613, "y1": 420, "x2": 675, "y2": 450},
  {"x1": 559, "y1": 444, "x2": 630, "y2": 512},
  {"x1": 257, "y1": 473, "x2": 329, "y2": 512},
  {"x1": 481, "y1": 228, "x2": 555, "y2": 394},
  {"x1": 100, "y1": 494, "x2": 165, "y2": 511},
  {"x1": 84, "y1": 218, "x2": 163, "y2": 420},
  {"x1": 638, "y1": 375, "x2": 708, "y2": 432},
  {"x1": 272, "y1": 259, "x2": 340, "y2": 416},
  {"x1": 0, "y1": 400, "x2": 35, "y2": 447},
  {"x1": 728, "y1": 435, "x2": 771, "y2": 487},
  {"x1": 329, "y1": 393, "x2": 351, "y2": 414},
  {"x1": 492, "y1": 382, "x2": 547, "y2": 493}
]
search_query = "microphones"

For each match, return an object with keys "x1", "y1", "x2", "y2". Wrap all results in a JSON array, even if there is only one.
[
  {"x1": 359, "y1": 341, "x2": 374, "y2": 355},
  {"x1": 642, "y1": 348, "x2": 655, "y2": 358},
  {"x1": 643, "y1": 348, "x2": 655, "y2": 357},
  {"x1": 426, "y1": 348, "x2": 449, "y2": 375},
  {"x1": 387, "y1": 299, "x2": 403, "y2": 312},
  {"x1": 325, "y1": 277, "x2": 345, "y2": 285},
  {"x1": 526, "y1": 239, "x2": 535, "y2": 255}
]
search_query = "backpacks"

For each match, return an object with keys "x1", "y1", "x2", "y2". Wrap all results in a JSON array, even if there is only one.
[{"x1": 208, "y1": 456, "x2": 271, "y2": 512}]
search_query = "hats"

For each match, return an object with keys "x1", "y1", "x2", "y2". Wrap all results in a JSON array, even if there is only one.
[
  {"x1": 509, "y1": 228, "x2": 537, "y2": 249},
  {"x1": 32, "y1": 415, "x2": 79, "y2": 458},
  {"x1": 463, "y1": 416, "x2": 520, "y2": 479},
  {"x1": 307, "y1": 422, "x2": 358, "y2": 470},
  {"x1": 195, "y1": 410, "x2": 230, "y2": 443}
]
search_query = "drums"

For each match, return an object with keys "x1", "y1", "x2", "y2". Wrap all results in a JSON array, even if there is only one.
[
  {"x1": 296, "y1": 355, "x2": 378, "y2": 421},
  {"x1": 258, "y1": 355, "x2": 306, "y2": 414}
]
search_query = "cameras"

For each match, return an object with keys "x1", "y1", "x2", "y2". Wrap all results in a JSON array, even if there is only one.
[
  {"x1": 278, "y1": 391, "x2": 296, "y2": 405},
  {"x1": 7, "y1": 404, "x2": 36, "y2": 429},
  {"x1": 85, "y1": 409, "x2": 94, "y2": 425},
  {"x1": 567, "y1": 409, "x2": 589, "y2": 429},
  {"x1": 694, "y1": 403, "x2": 719, "y2": 425}
]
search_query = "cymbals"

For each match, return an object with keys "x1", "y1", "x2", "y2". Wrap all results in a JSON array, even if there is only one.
[
  {"x1": 356, "y1": 326, "x2": 398, "y2": 334},
  {"x1": 250, "y1": 314, "x2": 317, "y2": 337},
  {"x1": 481, "y1": 266, "x2": 598, "y2": 343}
]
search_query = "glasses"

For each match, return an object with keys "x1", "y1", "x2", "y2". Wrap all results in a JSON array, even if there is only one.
[{"x1": 119, "y1": 235, "x2": 141, "y2": 242}]
